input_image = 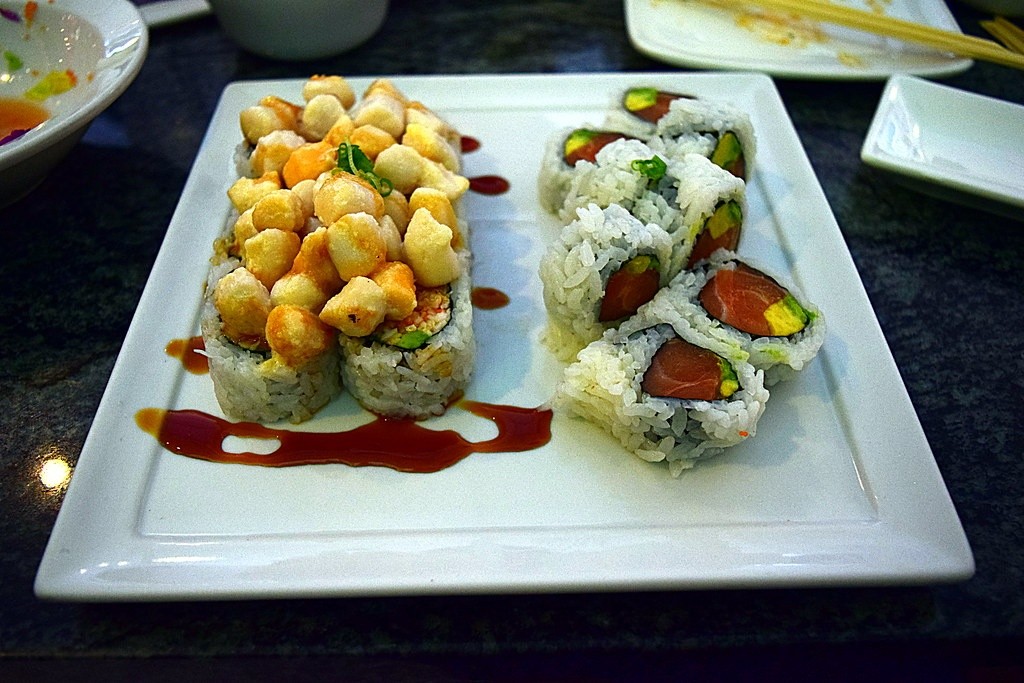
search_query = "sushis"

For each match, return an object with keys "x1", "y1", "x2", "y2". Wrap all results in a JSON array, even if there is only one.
[
  {"x1": 539, "y1": 83, "x2": 826, "y2": 477},
  {"x1": 197, "y1": 136, "x2": 475, "y2": 421}
]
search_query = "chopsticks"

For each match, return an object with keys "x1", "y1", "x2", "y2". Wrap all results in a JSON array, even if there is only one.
[{"x1": 761, "y1": 0, "x2": 1024, "y2": 71}]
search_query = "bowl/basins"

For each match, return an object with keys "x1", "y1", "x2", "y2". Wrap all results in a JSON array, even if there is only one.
[{"x1": 0, "y1": 0, "x2": 151, "y2": 170}]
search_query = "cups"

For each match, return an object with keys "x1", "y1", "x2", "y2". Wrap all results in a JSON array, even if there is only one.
[{"x1": 205, "y1": 0, "x2": 389, "y2": 59}]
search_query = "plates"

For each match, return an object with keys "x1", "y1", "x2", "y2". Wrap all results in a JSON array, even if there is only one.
[
  {"x1": 861, "y1": 74, "x2": 1024, "y2": 209},
  {"x1": 624, "y1": 1, "x2": 973, "y2": 79},
  {"x1": 35, "y1": 74, "x2": 976, "y2": 602}
]
